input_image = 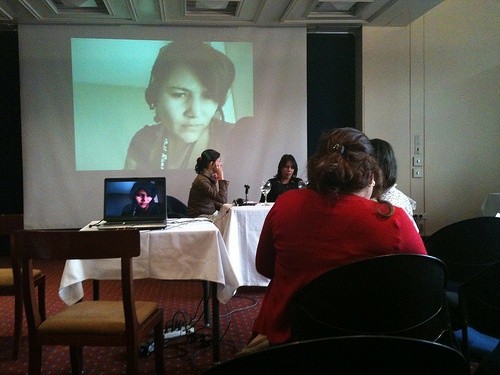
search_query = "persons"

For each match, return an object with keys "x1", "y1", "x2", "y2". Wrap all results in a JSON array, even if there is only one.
[
  {"x1": 123, "y1": 41, "x2": 236, "y2": 170},
  {"x1": 121, "y1": 182, "x2": 159, "y2": 217},
  {"x1": 370, "y1": 139, "x2": 420, "y2": 233},
  {"x1": 187, "y1": 150, "x2": 230, "y2": 217},
  {"x1": 259, "y1": 154, "x2": 303, "y2": 202},
  {"x1": 252, "y1": 128, "x2": 428, "y2": 346}
]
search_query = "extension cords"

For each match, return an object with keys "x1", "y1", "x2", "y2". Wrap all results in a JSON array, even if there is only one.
[{"x1": 163, "y1": 325, "x2": 194, "y2": 339}]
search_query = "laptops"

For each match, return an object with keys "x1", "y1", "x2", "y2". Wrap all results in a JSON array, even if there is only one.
[{"x1": 98, "y1": 177, "x2": 167, "y2": 231}]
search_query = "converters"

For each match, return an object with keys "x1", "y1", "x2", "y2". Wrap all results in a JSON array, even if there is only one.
[{"x1": 140, "y1": 337, "x2": 155, "y2": 353}]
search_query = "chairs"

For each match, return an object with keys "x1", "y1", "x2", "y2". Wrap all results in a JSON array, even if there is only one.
[
  {"x1": 166, "y1": 195, "x2": 197, "y2": 218},
  {"x1": 13, "y1": 228, "x2": 165, "y2": 375},
  {"x1": 0, "y1": 268, "x2": 46, "y2": 361},
  {"x1": 203, "y1": 217, "x2": 500, "y2": 375}
]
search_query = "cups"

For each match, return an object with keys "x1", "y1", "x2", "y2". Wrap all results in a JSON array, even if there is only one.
[{"x1": 298, "y1": 180, "x2": 307, "y2": 188}]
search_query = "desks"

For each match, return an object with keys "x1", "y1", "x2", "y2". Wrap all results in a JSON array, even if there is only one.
[
  {"x1": 216, "y1": 202, "x2": 275, "y2": 296},
  {"x1": 57, "y1": 217, "x2": 240, "y2": 366}
]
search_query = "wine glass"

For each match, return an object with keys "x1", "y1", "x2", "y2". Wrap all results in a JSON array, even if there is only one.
[{"x1": 260, "y1": 180, "x2": 271, "y2": 206}]
